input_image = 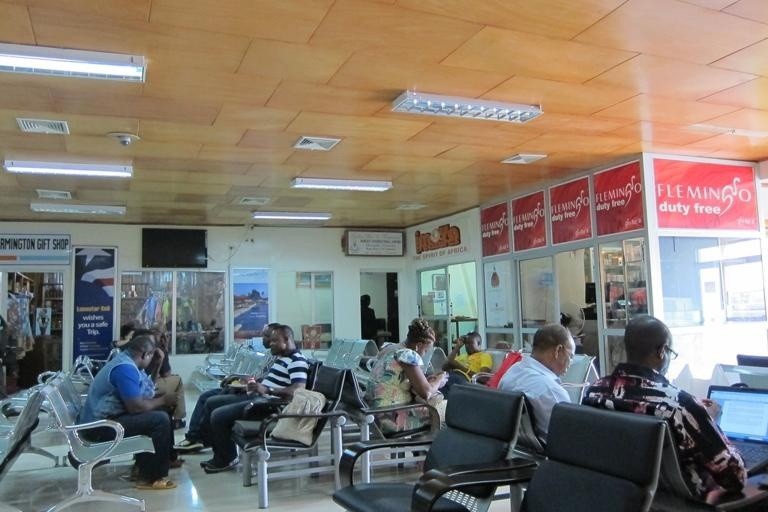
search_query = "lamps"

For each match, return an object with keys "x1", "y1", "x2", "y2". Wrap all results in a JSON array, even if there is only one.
[
  {"x1": 2, "y1": 159, "x2": 135, "y2": 179},
  {"x1": 251, "y1": 210, "x2": 332, "y2": 221},
  {"x1": 29, "y1": 202, "x2": 127, "y2": 217},
  {"x1": 0, "y1": 42, "x2": 146, "y2": 84},
  {"x1": 289, "y1": 176, "x2": 393, "y2": 192},
  {"x1": 391, "y1": 90, "x2": 544, "y2": 124}
]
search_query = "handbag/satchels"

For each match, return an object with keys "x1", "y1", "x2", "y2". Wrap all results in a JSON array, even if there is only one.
[{"x1": 270, "y1": 388, "x2": 326, "y2": 446}]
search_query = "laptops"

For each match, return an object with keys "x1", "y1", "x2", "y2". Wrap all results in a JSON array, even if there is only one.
[{"x1": 704, "y1": 385, "x2": 768, "y2": 477}]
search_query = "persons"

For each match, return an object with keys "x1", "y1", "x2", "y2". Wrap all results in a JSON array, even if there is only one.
[
  {"x1": 172, "y1": 323, "x2": 281, "y2": 450},
  {"x1": 498, "y1": 323, "x2": 577, "y2": 445},
  {"x1": 628, "y1": 296, "x2": 648, "y2": 314},
  {"x1": 441, "y1": 332, "x2": 492, "y2": 394},
  {"x1": 362, "y1": 294, "x2": 376, "y2": 337},
  {"x1": 79, "y1": 321, "x2": 187, "y2": 489},
  {"x1": 584, "y1": 314, "x2": 747, "y2": 506},
  {"x1": 200, "y1": 327, "x2": 309, "y2": 473},
  {"x1": 37, "y1": 308, "x2": 50, "y2": 335},
  {"x1": 366, "y1": 317, "x2": 450, "y2": 435},
  {"x1": 7, "y1": 297, "x2": 25, "y2": 339}
]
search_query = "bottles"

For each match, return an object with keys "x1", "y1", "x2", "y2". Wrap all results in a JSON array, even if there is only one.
[
  {"x1": 471, "y1": 376, "x2": 476, "y2": 385},
  {"x1": 247, "y1": 377, "x2": 254, "y2": 393}
]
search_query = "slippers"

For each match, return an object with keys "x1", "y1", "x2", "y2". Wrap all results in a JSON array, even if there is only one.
[{"x1": 136, "y1": 479, "x2": 177, "y2": 490}]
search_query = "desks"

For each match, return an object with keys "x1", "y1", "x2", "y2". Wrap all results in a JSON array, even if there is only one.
[{"x1": 420, "y1": 315, "x2": 478, "y2": 357}]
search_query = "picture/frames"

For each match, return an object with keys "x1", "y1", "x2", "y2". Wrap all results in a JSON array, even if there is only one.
[
  {"x1": 432, "y1": 273, "x2": 450, "y2": 290},
  {"x1": 229, "y1": 266, "x2": 276, "y2": 353}
]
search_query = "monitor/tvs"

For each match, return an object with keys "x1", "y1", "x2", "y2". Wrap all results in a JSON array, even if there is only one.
[{"x1": 142, "y1": 227, "x2": 208, "y2": 268}]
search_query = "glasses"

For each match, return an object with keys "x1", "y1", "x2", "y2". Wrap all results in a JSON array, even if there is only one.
[{"x1": 667, "y1": 346, "x2": 678, "y2": 360}]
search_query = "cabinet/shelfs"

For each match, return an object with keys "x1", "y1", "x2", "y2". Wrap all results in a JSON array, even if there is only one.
[
  {"x1": 41, "y1": 282, "x2": 63, "y2": 330},
  {"x1": 8, "y1": 272, "x2": 34, "y2": 295}
]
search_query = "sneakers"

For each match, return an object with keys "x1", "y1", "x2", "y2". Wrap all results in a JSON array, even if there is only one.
[
  {"x1": 201, "y1": 457, "x2": 239, "y2": 474},
  {"x1": 172, "y1": 420, "x2": 186, "y2": 429},
  {"x1": 173, "y1": 440, "x2": 213, "y2": 454}
]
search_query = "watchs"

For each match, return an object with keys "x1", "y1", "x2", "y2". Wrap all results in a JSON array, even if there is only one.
[{"x1": 266, "y1": 385, "x2": 274, "y2": 395}]
